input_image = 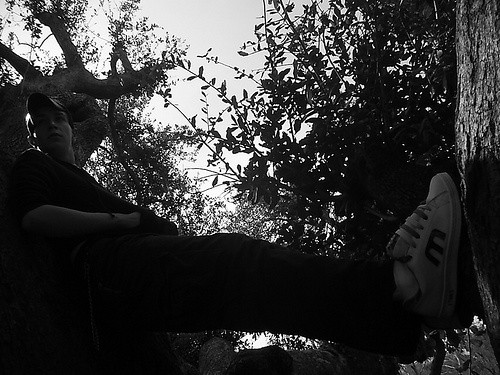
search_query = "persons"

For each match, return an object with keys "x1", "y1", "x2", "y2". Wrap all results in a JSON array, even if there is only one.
[{"x1": 8, "y1": 90, "x2": 476, "y2": 367}]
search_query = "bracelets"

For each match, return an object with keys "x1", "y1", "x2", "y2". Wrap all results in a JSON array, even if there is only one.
[{"x1": 110, "y1": 211, "x2": 123, "y2": 231}]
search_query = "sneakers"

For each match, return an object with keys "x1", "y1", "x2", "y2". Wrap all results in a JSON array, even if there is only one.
[{"x1": 386, "y1": 171, "x2": 461, "y2": 320}]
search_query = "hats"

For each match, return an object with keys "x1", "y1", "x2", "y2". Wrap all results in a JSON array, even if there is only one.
[{"x1": 27, "y1": 92, "x2": 74, "y2": 131}]
